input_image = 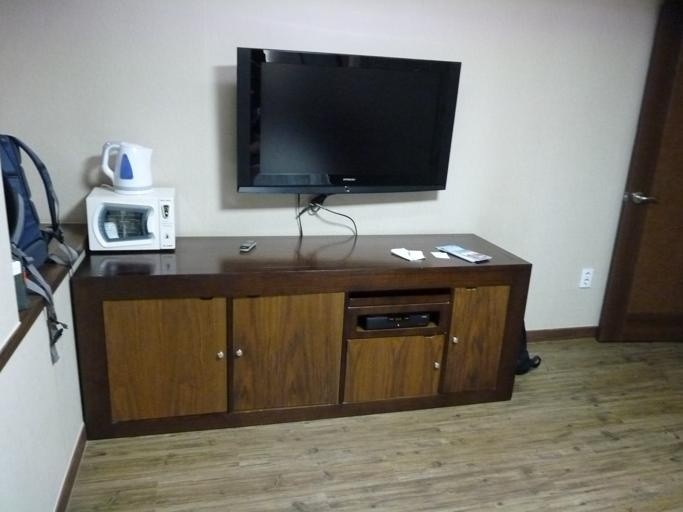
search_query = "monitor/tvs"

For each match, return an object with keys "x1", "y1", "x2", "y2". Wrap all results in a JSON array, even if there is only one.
[{"x1": 236, "y1": 44, "x2": 464, "y2": 196}]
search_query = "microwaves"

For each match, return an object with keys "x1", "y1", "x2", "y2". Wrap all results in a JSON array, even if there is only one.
[{"x1": 85, "y1": 186, "x2": 176, "y2": 251}]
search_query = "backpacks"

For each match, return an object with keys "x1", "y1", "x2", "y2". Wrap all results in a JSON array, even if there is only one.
[{"x1": 0, "y1": 135, "x2": 63, "y2": 276}]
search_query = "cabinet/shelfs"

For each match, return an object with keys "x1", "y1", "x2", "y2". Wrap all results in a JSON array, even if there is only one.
[{"x1": 71, "y1": 236, "x2": 533, "y2": 442}]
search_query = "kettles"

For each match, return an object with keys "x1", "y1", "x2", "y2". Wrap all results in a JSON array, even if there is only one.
[{"x1": 100, "y1": 142, "x2": 155, "y2": 195}]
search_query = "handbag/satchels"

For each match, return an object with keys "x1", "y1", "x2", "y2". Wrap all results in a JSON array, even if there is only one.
[{"x1": 515, "y1": 323, "x2": 541, "y2": 374}]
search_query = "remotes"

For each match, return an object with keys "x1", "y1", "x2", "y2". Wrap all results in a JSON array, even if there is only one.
[{"x1": 240, "y1": 238, "x2": 256, "y2": 253}]
